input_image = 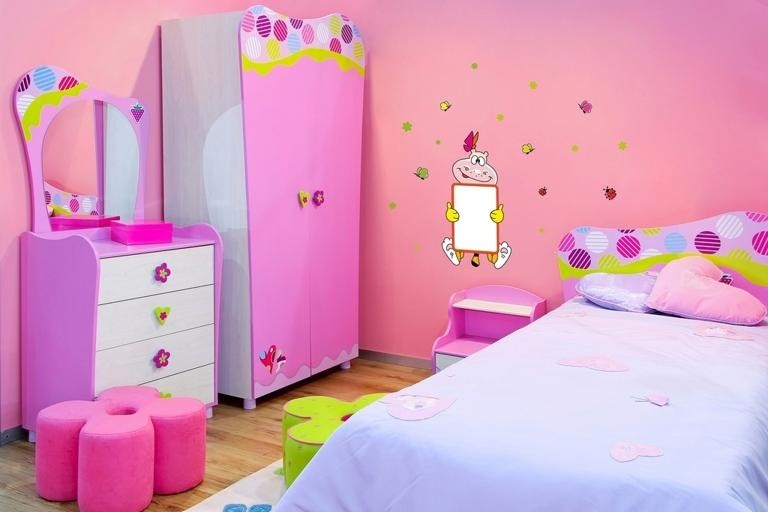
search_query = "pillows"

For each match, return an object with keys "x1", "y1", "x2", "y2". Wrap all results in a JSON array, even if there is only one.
[
  {"x1": 646, "y1": 256, "x2": 767, "y2": 326},
  {"x1": 576, "y1": 271, "x2": 658, "y2": 313},
  {"x1": 271, "y1": 208, "x2": 768, "y2": 511}
]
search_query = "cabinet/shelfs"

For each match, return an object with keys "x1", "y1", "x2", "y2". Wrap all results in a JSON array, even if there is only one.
[
  {"x1": 161, "y1": 4, "x2": 366, "y2": 409},
  {"x1": 18, "y1": 231, "x2": 225, "y2": 444}
]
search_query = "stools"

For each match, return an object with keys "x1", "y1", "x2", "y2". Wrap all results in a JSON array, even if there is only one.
[
  {"x1": 35, "y1": 386, "x2": 206, "y2": 512},
  {"x1": 283, "y1": 391, "x2": 390, "y2": 490}
]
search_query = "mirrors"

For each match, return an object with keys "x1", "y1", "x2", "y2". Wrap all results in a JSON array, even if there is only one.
[{"x1": 14, "y1": 64, "x2": 149, "y2": 242}]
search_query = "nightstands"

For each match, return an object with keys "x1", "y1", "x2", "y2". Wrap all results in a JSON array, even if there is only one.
[{"x1": 430, "y1": 284, "x2": 546, "y2": 375}]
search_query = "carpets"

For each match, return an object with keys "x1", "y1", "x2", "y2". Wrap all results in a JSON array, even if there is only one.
[{"x1": 182, "y1": 454, "x2": 288, "y2": 512}]
